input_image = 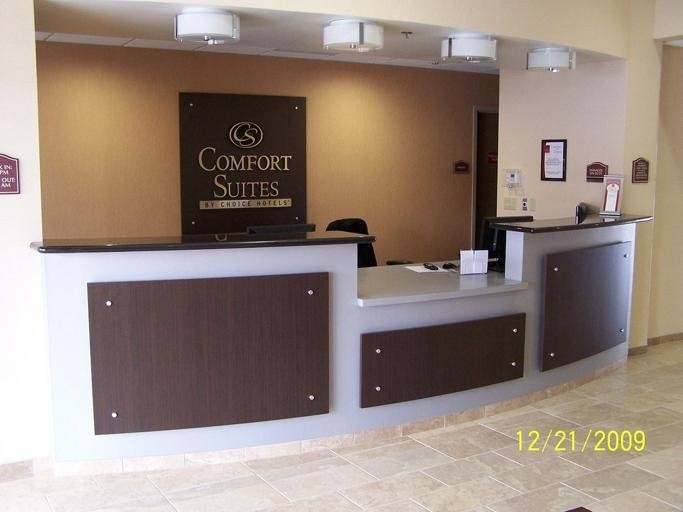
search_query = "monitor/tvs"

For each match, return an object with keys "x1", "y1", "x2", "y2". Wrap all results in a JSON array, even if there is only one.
[
  {"x1": 244, "y1": 223, "x2": 316, "y2": 234},
  {"x1": 479, "y1": 215, "x2": 534, "y2": 272},
  {"x1": 574, "y1": 216, "x2": 584, "y2": 224},
  {"x1": 575, "y1": 204, "x2": 587, "y2": 215}
]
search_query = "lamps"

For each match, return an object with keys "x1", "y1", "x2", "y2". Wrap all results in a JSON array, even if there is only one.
[
  {"x1": 437, "y1": 32, "x2": 500, "y2": 63},
  {"x1": 323, "y1": 18, "x2": 386, "y2": 54},
  {"x1": 171, "y1": 6, "x2": 242, "y2": 48},
  {"x1": 523, "y1": 45, "x2": 576, "y2": 72}
]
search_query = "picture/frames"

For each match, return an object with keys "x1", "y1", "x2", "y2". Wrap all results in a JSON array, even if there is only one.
[{"x1": 540, "y1": 140, "x2": 566, "y2": 182}]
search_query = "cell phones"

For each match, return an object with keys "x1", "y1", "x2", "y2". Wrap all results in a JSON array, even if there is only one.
[{"x1": 424, "y1": 262, "x2": 438, "y2": 270}]
